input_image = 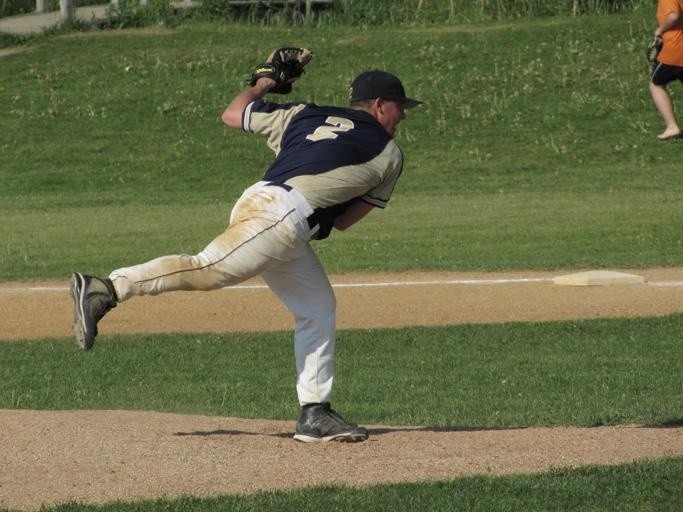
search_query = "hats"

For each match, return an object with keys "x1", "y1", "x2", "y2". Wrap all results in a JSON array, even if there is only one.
[{"x1": 349, "y1": 67, "x2": 424, "y2": 115}]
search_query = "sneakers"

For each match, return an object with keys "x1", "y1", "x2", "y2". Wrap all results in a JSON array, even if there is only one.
[
  {"x1": 293, "y1": 402, "x2": 370, "y2": 444},
  {"x1": 69, "y1": 271, "x2": 117, "y2": 354}
]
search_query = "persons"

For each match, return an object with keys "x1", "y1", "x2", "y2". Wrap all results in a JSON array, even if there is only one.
[
  {"x1": 649, "y1": 0, "x2": 683, "y2": 140},
  {"x1": 68, "y1": 46, "x2": 427, "y2": 445}
]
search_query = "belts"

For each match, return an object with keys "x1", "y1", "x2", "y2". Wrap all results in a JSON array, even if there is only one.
[{"x1": 264, "y1": 182, "x2": 320, "y2": 239}]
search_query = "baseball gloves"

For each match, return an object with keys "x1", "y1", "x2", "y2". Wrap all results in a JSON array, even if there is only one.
[
  {"x1": 646, "y1": 37, "x2": 663, "y2": 62},
  {"x1": 250, "y1": 47, "x2": 312, "y2": 94}
]
